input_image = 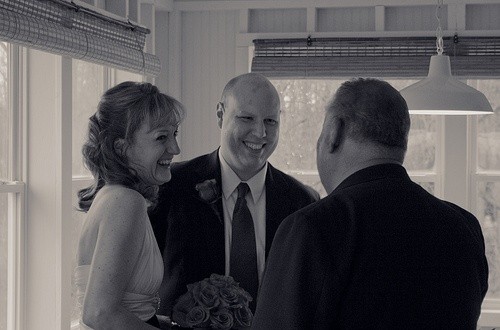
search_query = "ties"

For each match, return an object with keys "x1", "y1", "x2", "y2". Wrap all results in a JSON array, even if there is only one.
[{"x1": 227, "y1": 180, "x2": 261, "y2": 322}]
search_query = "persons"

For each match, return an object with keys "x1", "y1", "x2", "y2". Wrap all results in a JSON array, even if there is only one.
[
  {"x1": 149, "y1": 72, "x2": 320, "y2": 330},
  {"x1": 70, "y1": 80, "x2": 181, "y2": 330},
  {"x1": 249, "y1": 77, "x2": 491, "y2": 330}
]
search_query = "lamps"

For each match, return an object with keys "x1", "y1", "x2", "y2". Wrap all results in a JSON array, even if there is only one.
[{"x1": 398, "y1": 0, "x2": 496, "y2": 116}]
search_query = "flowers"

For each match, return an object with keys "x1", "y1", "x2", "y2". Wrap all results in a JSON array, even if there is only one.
[{"x1": 171, "y1": 273, "x2": 254, "y2": 330}]
